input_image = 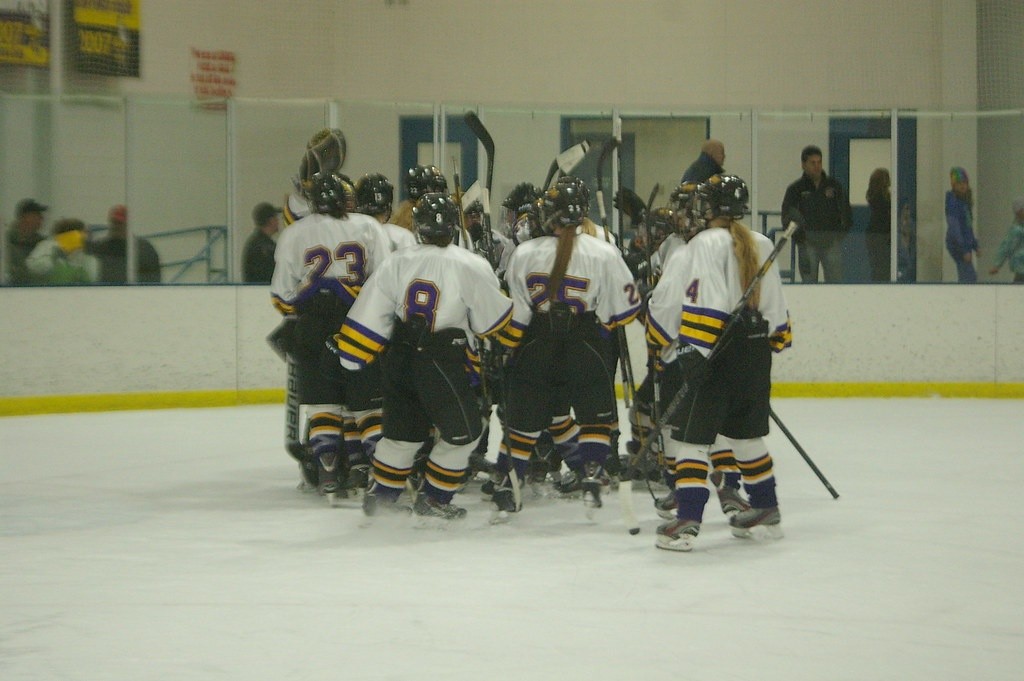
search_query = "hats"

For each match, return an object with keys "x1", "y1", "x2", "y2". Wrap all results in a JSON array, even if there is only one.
[
  {"x1": 252, "y1": 203, "x2": 283, "y2": 225},
  {"x1": 16, "y1": 198, "x2": 48, "y2": 212}
]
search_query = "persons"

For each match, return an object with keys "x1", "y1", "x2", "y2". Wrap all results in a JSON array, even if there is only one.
[
  {"x1": 240, "y1": 202, "x2": 283, "y2": 284},
  {"x1": 866, "y1": 168, "x2": 892, "y2": 282},
  {"x1": 944, "y1": 167, "x2": 982, "y2": 284},
  {"x1": 681, "y1": 137, "x2": 726, "y2": 184},
  {"x1": 0, "y1": 197, "x2": 161, "y2": 287},
  {"x1": 989, "y1": 196, "x2": 1024, "y2": 283},
  {"x1": 271, "y1": 122, "x2": 791, "y2": 539},
  {"x1": 782, "y1": 145, "x2": 852, "y2": 283}
]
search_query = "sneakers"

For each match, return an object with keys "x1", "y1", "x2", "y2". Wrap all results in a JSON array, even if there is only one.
[
  {"x1": 296, "y1": 431, "x2": 620, "y2": 527},
  {"x1": 710, "y1": 470, "x2": 750, "y2": 518},
  {"x1": 730, "y1": 506, "x2": 784, "y2": 539},
  {"x1": 655, "y1": 490, "x2": 678, "y2": 520},
  {"x1": 626, "y1": 441, "x2": 666, "y2": 489},
  {"x1": 655, "y1": 519, "x2": 700, "y2": 552}
]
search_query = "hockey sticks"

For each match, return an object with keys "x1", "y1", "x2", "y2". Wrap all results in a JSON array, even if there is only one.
[
  {"x1": 281, "y1": 105, "x2": 841, "y2": 526},
  {"x1": 617, "y1": 220, "x2": 799, "y2": 536}
]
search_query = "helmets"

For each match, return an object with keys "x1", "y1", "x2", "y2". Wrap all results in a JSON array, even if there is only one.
[
  {"x1": 697, "y1": 174, "x2": 751, "y2": 219},
  {"x1": 355, "y1": 172, "x2": 394, "y2": 214},
  {"x1": 404, "y1": 164, "x2": 447, "y2": 202},
  {"x1": 670, "y1": 181, "x2": 707, "y2": 226},
  {"x1": 413, "y1": 193, "x2": 456, "y2": 236},
  {"x1": 538, "y1": 176, "x2": 591, "y2": 234},
  {"x1": 501, "y1": 182, "x2": 542, "y2": 238},
  {"x1": 649, "y1": 207, "x2": 675, "y2": 233},
  {"x1": 309, "y1": 172, "x2": 355, "y2": 212}
]
